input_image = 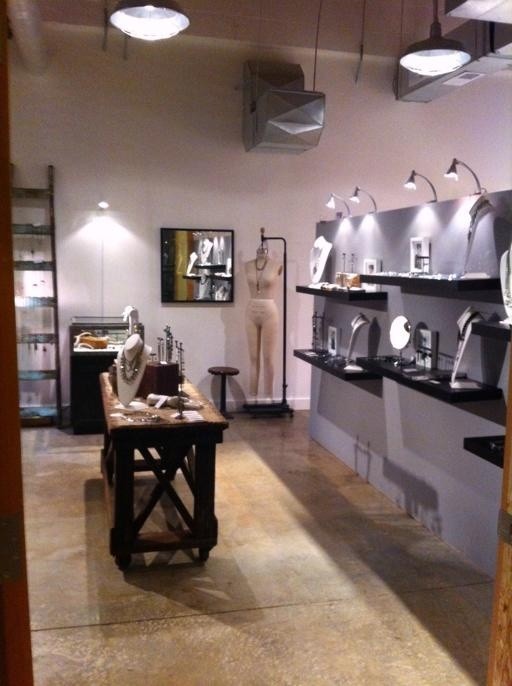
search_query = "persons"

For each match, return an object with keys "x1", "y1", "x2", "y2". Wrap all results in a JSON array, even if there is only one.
[{"x1": 244, "y1": 247, "x2": 283, "y2": 399}]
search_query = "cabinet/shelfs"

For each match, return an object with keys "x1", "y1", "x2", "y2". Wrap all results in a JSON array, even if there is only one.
[{"x1": 290, "y1": 272, "x2": 512, "y2": 468}]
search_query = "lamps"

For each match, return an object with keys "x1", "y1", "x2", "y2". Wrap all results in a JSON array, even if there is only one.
[
  {"x1": 325, "y1": 153, "x2": 483, "y2": 218},
  {"x1": 399, "y1": 0, "x2": 472, "y2": 79}
]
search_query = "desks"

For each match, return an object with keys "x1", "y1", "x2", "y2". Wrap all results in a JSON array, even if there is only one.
[
  {"x1": 68, "y1": 345, "x2": 124, "y2": 435},
  {"x1": 97, "y1": 366, "x2": 230, "y2": 574}
]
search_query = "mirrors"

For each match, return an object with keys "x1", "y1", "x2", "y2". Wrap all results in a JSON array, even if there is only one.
[{"x1": 160, "y1": 224, "x2": 235, "y2": 304}]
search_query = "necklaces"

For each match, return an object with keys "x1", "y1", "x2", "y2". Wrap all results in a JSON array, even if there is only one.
[
  {"x1": 201, "y1": 245, "x2": 210, "y2": 254},
  {"x1": 466, "y1": 197, "x2": 489, "y2": 240},
  {"x1": 118, "y1": 342, "x2": 143, "y2": 384},
  {"x1": 456, "y1": 311, "x2": 481, "y2": 340},
  {"x1": 199, "y1": 277, "x2": 208, "y2": 285},
  {"x1": 253, "y1": 258, "x2": 269, "y2": 272},
  {"x1": 502, "y1": 250, "x2": 512, "y2": 306}
]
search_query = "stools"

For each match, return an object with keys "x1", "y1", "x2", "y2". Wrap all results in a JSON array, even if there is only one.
[{"x1": 207, "y1": 365, "x2": 240, "y2": 422}]
